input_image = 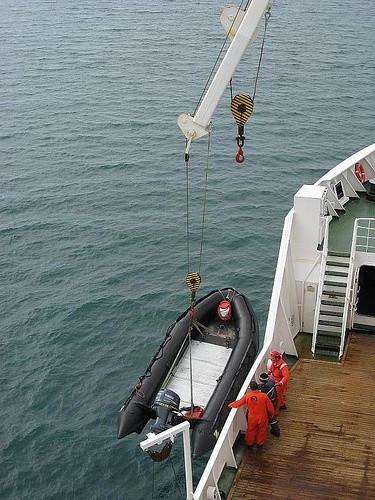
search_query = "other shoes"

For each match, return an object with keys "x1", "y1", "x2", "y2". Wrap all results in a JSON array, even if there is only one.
[{"x1": 280, "y1": 404, "x2": 286, "y2": 409}]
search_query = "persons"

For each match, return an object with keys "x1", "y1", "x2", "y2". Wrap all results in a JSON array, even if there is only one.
[
  {"x1": 227, "y1": 382, "x2": 275, "y2": 452},
  {"x1": 266, "y1": 351, "x2": 290, "y2": 410},
  {"x1": 255, "y1": 372, "x2": 280, "y2": 437}
]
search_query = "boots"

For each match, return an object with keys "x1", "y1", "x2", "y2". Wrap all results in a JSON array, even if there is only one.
[
  {"x1": 248, "y1": 445, "x2": 254, "y2": 452},
  {"x1": 270, "y1": 420, "x2": 281, "y2": 436},
  {"x1": 257, "y1": 445, "x2": 264, "y2": 455}
]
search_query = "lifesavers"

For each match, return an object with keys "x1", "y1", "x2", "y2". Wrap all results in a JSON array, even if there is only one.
[{"x1": 355, "y1": 162, "x2": 366, "y2": 183}]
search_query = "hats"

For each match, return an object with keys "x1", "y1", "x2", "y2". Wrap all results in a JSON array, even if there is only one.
[{"x1": 271, "y1": 351, "x2": 281, "y2": 357}]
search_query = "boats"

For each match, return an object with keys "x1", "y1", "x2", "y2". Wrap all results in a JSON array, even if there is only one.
[{"x1": 116, "y1": 286, "x2": 260, "y2": 460}]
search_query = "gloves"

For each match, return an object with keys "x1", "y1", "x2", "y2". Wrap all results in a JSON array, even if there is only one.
[{"x1": 275, "y1": 381, "x2": 282, "y2": 387}]
